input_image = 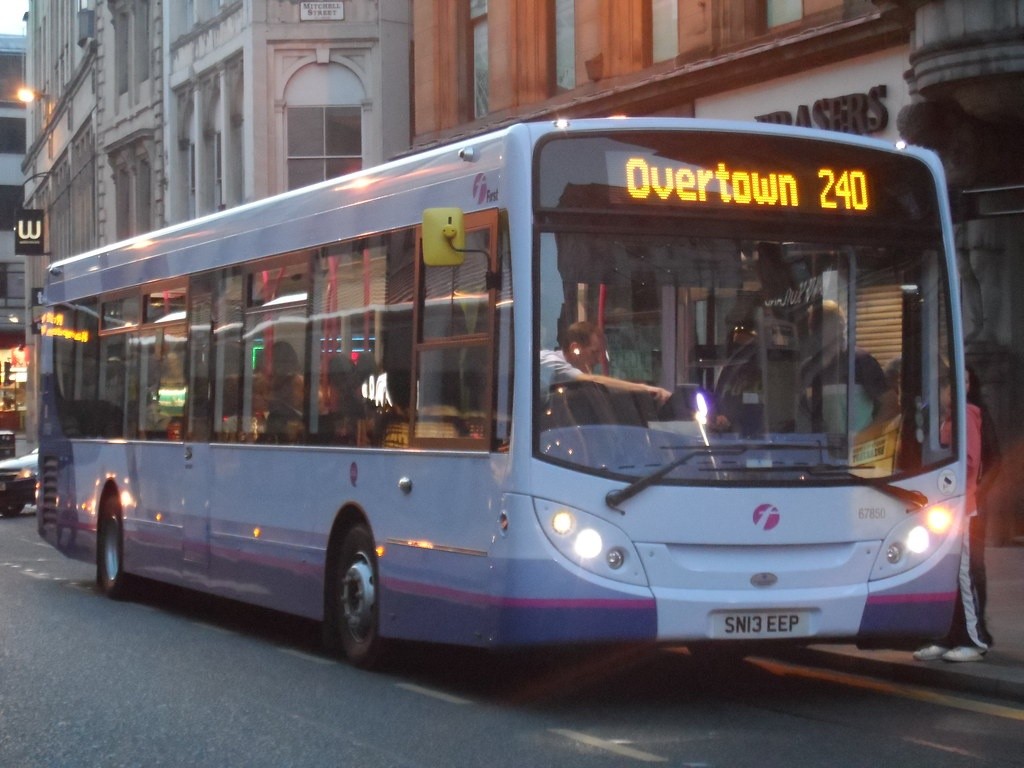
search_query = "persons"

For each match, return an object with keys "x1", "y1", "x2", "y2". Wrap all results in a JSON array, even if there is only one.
[
  {"x1": 538, "y1": 320, "x2": 673, "y2": 408},
  {"x1": 912, "y1": 363, "x2": 1001, "y2": 663},
  {"x1": 222, "y1": 342, "x2": 374, "y2": 444},
  {"x1": 715, "y1": 324, "x2": 801, "y2": 433},
  {"x1": 796, "y1": 300, "x2": 898, "y2": 459}
]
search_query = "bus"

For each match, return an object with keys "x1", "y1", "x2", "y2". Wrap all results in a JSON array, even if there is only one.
[{"x1": 23, "y1": 113, "x2": 973, "y2": 676}]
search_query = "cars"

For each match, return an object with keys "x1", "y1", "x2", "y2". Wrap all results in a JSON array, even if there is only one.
[{"x1": 0, "y1": 447, "x2": 40, "y2": 517}]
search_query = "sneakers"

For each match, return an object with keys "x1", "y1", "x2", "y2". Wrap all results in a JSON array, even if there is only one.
[
  {"x1": 913, "y1": 645, "x2": 950, "y2": 661},
  {"x1": 942, "y1": 645, "x2": 985, "y2": 661}
]
